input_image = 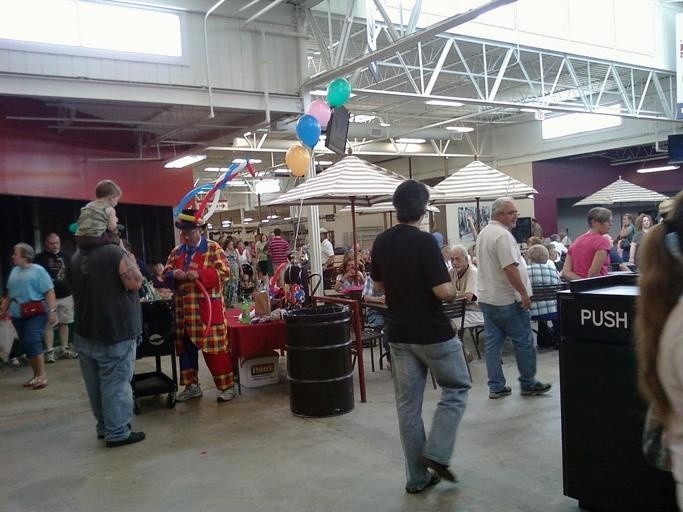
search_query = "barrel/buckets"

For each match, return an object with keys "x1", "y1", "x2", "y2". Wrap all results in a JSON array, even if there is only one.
[{"x1": 280, "y1": 305, "x2": 356, "y2": 417}]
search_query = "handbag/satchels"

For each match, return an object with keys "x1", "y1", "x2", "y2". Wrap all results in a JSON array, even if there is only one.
[{"x1": 19, "y1": 301, "x2": 46, "y2": 319}]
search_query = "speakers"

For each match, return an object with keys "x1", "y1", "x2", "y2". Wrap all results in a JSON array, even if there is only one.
[{"x1": 555, "y1": 285, "x2": 680, "y2": 512}]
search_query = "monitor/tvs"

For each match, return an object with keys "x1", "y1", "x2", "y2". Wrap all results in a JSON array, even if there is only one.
[
  {"x1": 325, "y1": 104, "x2": 350, "y2": 157},
  {"x1": 667, "y1": 134, "x2": 683, "y2": 165},
  {"x1": 516, "y1": 217, "x2": 531, "y2": 243}
]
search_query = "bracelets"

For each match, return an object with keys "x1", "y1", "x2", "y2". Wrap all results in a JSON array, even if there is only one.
[{"x1": 48, "y1": 307, "x2": 56, "y2": 312}]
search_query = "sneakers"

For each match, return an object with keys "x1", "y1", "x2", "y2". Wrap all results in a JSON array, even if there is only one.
[
  {"x1": 59, "y1": 345, "x2": 79, "y2": 359},
  {"x1": 418, "y1": 457, "x2": 454, "y2": 483},
  {"x1": 22, "y1": 375, "x2": 48, "y2": 389},
  {"x1": 98, "y1": 422, "x2": 145, "y2": 448},
  {"x1": 216, "y1": 383, "x2": 239, "y2": 402},
  {"x1": 489, "y1": 386, "x2": 512, "y2": 399},
  {"x1": 521, "y1": 384, "x2": 551, "y2": 395},
  {"x1": 45, "y1": 348, "x2": 56, "y2": 363},
  {"x1": 176, "y1": 383, "x2": 202, "y2": 402},
  {"x1": 405, "y1": 471, "x2": 440, "y2": 494}
]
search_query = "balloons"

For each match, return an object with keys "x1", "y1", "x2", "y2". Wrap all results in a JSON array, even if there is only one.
[
  {"x1": 174, "y1": 159, "x2": 255, "y2": 223},
  {"x1": 328, "y1": 79, "x2": 350, "y2": 110},
  {"x1": 296, "y1": 115, "x2": 321, "y2": 148},
  {"x1": 308, "y1": 99, "x2": 332, "y2": 130},
  {"x1": 284, "y1": 144, "x2": 311, "y2": 177}
]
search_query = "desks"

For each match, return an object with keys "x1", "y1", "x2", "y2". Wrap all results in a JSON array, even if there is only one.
[{"x1": 223, "y1": 308, "x2": 289, "y2": 395}]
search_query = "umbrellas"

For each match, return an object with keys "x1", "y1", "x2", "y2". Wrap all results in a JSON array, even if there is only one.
[
  {"x1": 433, "y1": 155, "x2": 536, "y2": 235},
  {"x1": 266, "y1": 149, "x2": 443, "y2": 287},
  {"x1": 341, "y1": 201, "x2": 441, "y2": 234}
]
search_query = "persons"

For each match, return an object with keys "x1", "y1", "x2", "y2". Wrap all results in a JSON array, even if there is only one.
[
  {"x1": 634, "y1": 192, "x2": 681, "y2": 511},
  {"x1": 32, "y1": 232, "x2": 79, "y2": 363},
  {"x1": 150, "y1": 207, "x2": 239, "y2": 402},
  {"x1": 68, "y1": 181, "x2": 145, "y2": 447},
  {"x1": 337, "y1": 243, "x2": 366, "y2": 317},
  {"x1": 362, "y1": 180, "x2": 484, "y2": 493},
  {"x1": 2, "y1": 242, "x2": 57, "y2": 389},
  {"x1": 1, "y1": 295, "x2": 26, "y2": 365},
  {"x1": 476, "y1": 197, "x2": 567, "y2": 399},
  {"x1": 439, "y1": 243, "x2": 477, "y2": 309},
  {"x1": 236, "y1": 228, "x2": 335, "y2": 286},
  {"x1": 560, "y1": 208, "x2": 653, "y2": 282}
]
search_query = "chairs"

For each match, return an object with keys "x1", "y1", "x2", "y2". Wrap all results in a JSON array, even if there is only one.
[{"x1": 323, "y1": 262, "x2": 640, "y2": 389}]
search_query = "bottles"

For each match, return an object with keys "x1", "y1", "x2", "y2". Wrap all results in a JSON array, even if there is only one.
[{"x1": 241, "y1": 296, "x2": 251, "y2": 325}]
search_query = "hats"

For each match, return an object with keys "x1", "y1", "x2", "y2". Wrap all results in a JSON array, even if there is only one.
[
  {"x1": 660, "y1": 199, "x2": 675, "y2": 215},
  {"x1": 175, "y1": 209, "x2": 207, "y2": 230}
]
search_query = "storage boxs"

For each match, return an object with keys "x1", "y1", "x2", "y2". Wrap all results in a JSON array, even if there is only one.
[{"x1": 237, "y1": 352, "x2": 280, "y2": 390}]
